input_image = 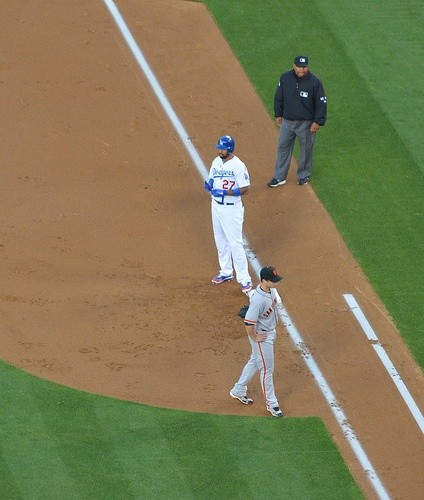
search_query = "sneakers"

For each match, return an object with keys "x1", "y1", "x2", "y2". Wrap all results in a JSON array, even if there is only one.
[
  {"x1": 296, "y1": 176, "x2": 310, "y2": 185},
  {"x1": 267, "y1": 405, "x2": 283, "y2": 417},
  {"x1": 268, "y1": 179, "x2": 286, "y2": 187},
  {"x1": 212, "y1": 273, "x2": 234, "y2": 283},
  {"x1": 241, "y1": 281, "x2": 252, "y2": 292},
  {"x1": 229, "y1": 391, "x2": 253, "y2": 405}
]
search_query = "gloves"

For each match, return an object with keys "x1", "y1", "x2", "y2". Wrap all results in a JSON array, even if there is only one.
[
  {"x1": 205, "y1": 179, "x2": 214, "y2": 191},
  {"x1": 211, "y1": 190, "x2": 227, "y2": 197}
]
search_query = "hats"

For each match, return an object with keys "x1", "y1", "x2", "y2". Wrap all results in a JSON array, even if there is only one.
[
  {"x1": 260, "y1": 267, "x2": 282, "y2": 283},
  {"x1": 295, "y1": 56, "x2": 309, "y2": 67}
]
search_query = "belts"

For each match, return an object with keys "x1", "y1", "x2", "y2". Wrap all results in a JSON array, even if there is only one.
[{"x1": 214, "y1": 199, "x2": 234, "y2": 205}]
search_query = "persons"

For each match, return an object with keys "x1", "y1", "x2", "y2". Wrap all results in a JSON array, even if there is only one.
[
  {"x1": 267, "y1": 56, "x2": 327, "y2": 188},
  {"x1": 230, "y1": 266, "x2": 284, "y2": 417},
  {"x1": 204, "y1": 134, "x2": 252, "y2": 292}
]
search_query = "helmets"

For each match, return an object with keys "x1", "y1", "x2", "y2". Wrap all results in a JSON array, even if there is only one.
[{"x1": 216, "y1": 135, "x2": 234, "y2": 155}]
